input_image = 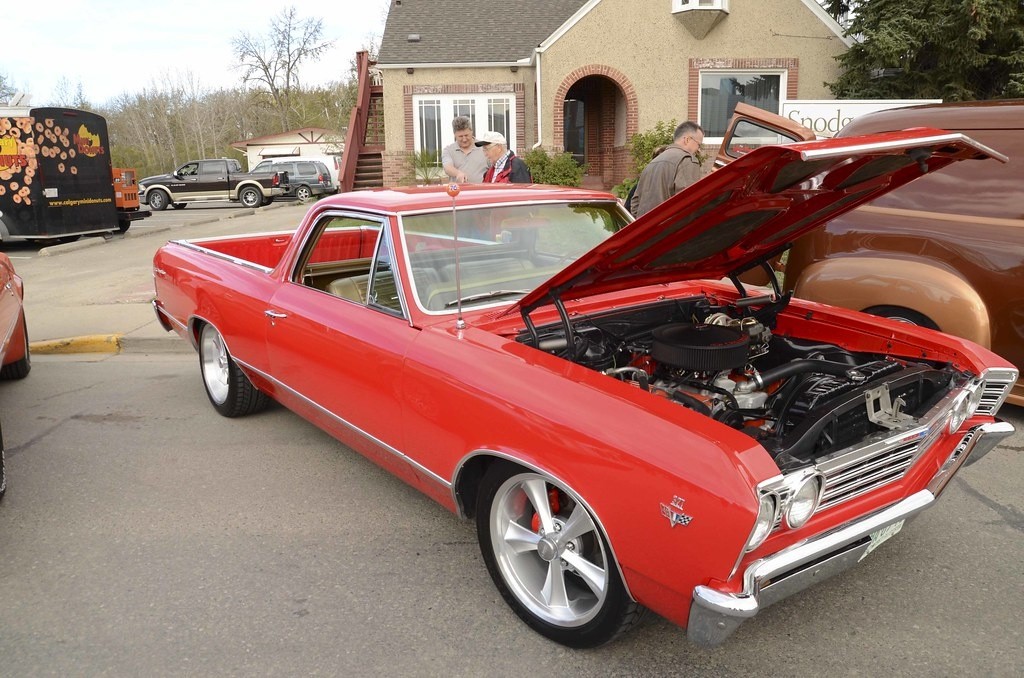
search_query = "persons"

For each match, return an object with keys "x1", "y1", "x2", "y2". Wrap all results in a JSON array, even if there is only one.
[
  {"x1": 442, "y1": 116, "x2": 488, "y2": 239},
  {"x1": 475, "y1": 132, "x2": 533, "y2": 241},
  {"x1": 625, "y1": 121, "x2": 706, "y2": 220}
]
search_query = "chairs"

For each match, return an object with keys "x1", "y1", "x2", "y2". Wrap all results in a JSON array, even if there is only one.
[{"x1": 327, "y1": 258, "x2": 537, "y2": 310}]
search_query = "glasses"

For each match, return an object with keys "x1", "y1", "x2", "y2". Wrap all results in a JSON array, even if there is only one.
[{"x1": 482, "y1": 143, "x2": 499, "y2": 151}]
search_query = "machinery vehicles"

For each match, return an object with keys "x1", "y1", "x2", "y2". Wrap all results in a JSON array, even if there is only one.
[{"x1": 110, "y1": 166, "x2": 153, "y2": 235}]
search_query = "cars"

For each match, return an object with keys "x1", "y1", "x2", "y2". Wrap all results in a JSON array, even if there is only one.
[
  {"x1": 151, "y1": 127, "x2": 1020, "y2": 651},
  {"x1": 703, "y1": 99, "x2": 1023, "y2": 412},
  {"x1": 1, "y1": 251, "x2": 31, "y2": 497}
]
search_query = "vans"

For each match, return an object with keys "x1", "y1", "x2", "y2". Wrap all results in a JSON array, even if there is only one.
[{"x1": 252, "y1": 155, "x2": 339, "y2": 192}]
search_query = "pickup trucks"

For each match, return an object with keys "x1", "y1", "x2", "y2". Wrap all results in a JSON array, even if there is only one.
[{"x1": 139, "y1": 156, "x2": 292, "y2": 211}]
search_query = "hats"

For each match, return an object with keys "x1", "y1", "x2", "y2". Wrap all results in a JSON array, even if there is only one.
[{"x1": 475, "y1": 130, "x2": 506, "y2": 147}]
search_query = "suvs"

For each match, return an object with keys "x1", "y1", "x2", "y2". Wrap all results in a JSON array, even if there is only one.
[{"x1": 247, "y1": 159, "x2": 335, "y2": 203}]
